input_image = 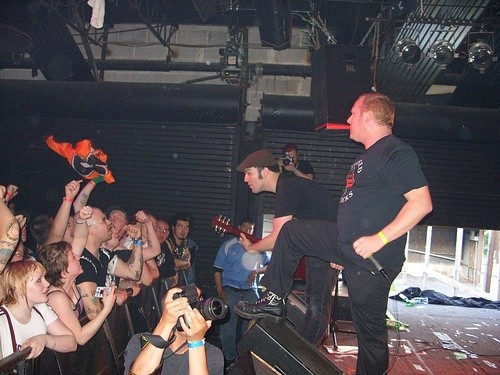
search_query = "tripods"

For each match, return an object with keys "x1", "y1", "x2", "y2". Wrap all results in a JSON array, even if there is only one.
[{"x1": 325, "y1": 270, "x2": 358, "y2": 350}]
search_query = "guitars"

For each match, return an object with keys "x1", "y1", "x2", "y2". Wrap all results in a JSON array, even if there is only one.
[{"x1": 211, "y1": 214, "x2": 306, "y2": 282}]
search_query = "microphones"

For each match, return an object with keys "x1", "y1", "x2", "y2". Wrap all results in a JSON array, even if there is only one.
[{"x1": 368, "y1": 255, "x2": 390, "y2": 281}]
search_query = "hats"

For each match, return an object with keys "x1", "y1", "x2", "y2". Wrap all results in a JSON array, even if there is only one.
[{"x1": 236, "y1": 149, "x2": 278, "y2": 172}]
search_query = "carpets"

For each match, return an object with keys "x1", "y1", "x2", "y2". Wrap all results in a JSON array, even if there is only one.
[{"x1": 294, "y1": 292, "x2": 390, "y2": 323}]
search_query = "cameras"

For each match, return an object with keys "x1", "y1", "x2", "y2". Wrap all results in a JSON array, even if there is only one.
[
  {"x1": 169, "y1": 284, "x2": 228, "y2": 331},
  {"x1": 95, "y1": 287, "x2": 112, "y2": 298},
  {"x1": 282, "y1": 158, "x2": 293, "y2": 166}
]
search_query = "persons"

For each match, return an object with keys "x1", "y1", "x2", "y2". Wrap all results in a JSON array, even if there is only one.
[
  {"x1": 124, "y1": 286, "x2": 224, "y2": 375},
  {"x1": 276, "y1": 143, "x2": 315, "y2": 181},
  {"x1": 233, "y1": 93, "x2": 432, "y2": 375},
  {"x1": 236, "y1": 149, "x2": 338, "y2": 345},
  {"x1": 212, "y1": 218, "x2": 270, "y2": 371},
  {"x1": 0, "y1": 171, "x2": 198, "y2": 375}
]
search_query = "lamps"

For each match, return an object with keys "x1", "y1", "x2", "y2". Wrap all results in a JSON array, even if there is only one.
[
  {"x1": 393, "y1": 22, "x2": 423, "y2": 67},
  {"x1": 466, "y1": 25, "x2": 495, "y2": 72},
  {"x1": 220, "y1": 41, "x2": 245, "y2": 87},
  {"x1": 428, "y1": 24, "x2": 456, "y2": 68}
]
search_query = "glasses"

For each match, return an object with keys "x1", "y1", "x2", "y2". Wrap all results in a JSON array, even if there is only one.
[
  {"x1": 176, "y1": 224, "x2": 190, "y2": 231},
  {"x1": 87, "y1": 216, "x2": 108, "y2": 228},
  {"x1": 156, "y1": 227, "x2": 170, "y2": 234}
]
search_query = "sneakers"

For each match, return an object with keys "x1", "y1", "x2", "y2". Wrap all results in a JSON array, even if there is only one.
[{"x1": 234, "y1": 295, "x2": 287, "y2": 324}]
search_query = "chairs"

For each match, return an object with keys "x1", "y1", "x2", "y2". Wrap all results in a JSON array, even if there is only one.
[{"x1": 0, "y1": 268, "x2": 189, "y2": 375}]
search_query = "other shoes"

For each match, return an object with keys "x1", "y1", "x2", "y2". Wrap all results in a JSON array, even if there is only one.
[{"x1": 224, "y1": 356, "x2": 240, "y2": 370}]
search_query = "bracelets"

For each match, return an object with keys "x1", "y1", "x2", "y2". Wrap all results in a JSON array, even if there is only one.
[
  {"x1": 187, "y1": 338, "x2": 206, "y2": 348},
  {"x1": 294, "y1": 169, "x2": 296, "y2": 172},
  {"x1": 378, "y1": 231, "x2": 388, "y2": 244}
]
